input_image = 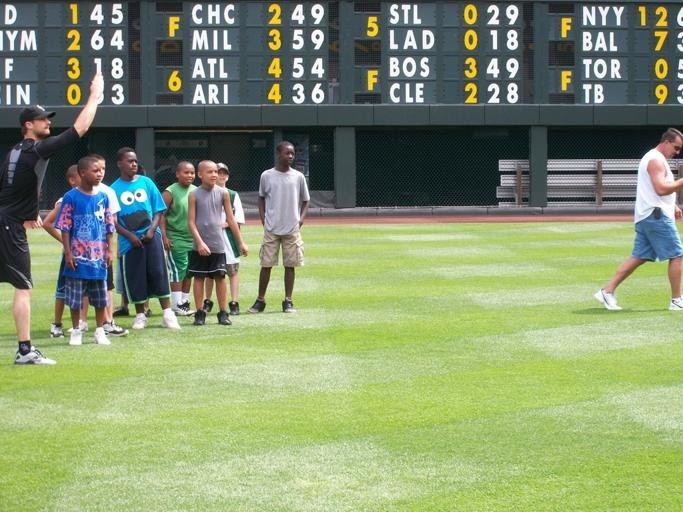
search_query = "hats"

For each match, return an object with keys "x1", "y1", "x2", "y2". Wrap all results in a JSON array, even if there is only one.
[
  {"x1": 19, "y1": 105, "x2": 56, "y2": 123},
  {"x1": 216, "y1": 163, "x2": 229, "y2": 174}
]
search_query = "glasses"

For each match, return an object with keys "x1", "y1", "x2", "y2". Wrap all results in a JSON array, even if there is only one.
[{"x1": 672, "y1": 144, "x2": 681, "y2": 152}]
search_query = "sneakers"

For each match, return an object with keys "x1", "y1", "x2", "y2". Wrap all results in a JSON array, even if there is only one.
[
  {"x1": 70, "y1": 330, "x2": 83, "y2": 345},
  {"x1": 161, "y1": 312, "x2": 181, "y2": 329},
  {"x1": 217, "y1": 312, "x2": 231, "y2": 325},
  {"x1": 113, "y1": 307, "x2": 129, "y2": 316},
  {"x1": 132, "y1": 316, "x2": 145, "y2": 329},
  {"x1": 171, "y1": 303, "x2": 195, "y2": 316},
  {"x1": 281, "y1": 299, "x2": 295, "y2": 313},
  {"x1": 669, "y1": 296, "x2": 683, "y2": 310},
  {"x1": 103, "y1": 321, "x2": 128, "y2": 337},
  {"x1": 229, "y1": 302, "x2": 238, "y2": 315},
  {"x1": 203, "y1": 299, "x2": 213, "y2": 312},
  {"x1": 194, "y1": 311, "x2": 206, "y2": 325},
  {"x1": 95, "y1": 329, "x2": 111, "y2": 345},
  {"x1": 247, "y1": 299, "x2": 265, "y2": 313},
  {"x1": 595, "y1": 289, "x2": 624, "y2": 310},
  {"x1": 67, "y1": 320, "x2": 89, "y2": 333},
  {"x1": 181, "y1": 301, "x2": 189, "y2": 309},
  {"x1": 15, "y1": 345, "x2": 57, "y2": 364},
  {"x1": 50, "y1": 323, "x2": 65, "y2": 338}
]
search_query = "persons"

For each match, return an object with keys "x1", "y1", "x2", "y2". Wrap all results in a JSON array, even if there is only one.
[
  {"x1": 247, "y1": 141, "x2": 311, "y2": 314},
  {"x1": 41, "y1": 164, "x2": 129, "y2": 339},
  {"x1": 202, "y1": 162, "x2": 245, "y2": 315},
  {"x1": 158, "y1": 161, "x2": 198, "y2": 317},
  {"x1": 108, "y1": 147, "x2": 181, "y2": 329},
  {"x1": 593, "y1": 127, "x2": 683, "y2": 313},
  {"x1": 187, "y1": 160, "x2": 247, "y2": 325},
  {"x1": 67, "y1": 154, "x2": 121, "y2": 334},
  {"x1": 53, "y1": 156, "x2": 116, "y2": 346},
  {"x1": 111, "y1": 162, "x2": 151, "y2": 318},
  {"x1": 0, "y1": 71, "x2": 103, "y2": 365}
]
